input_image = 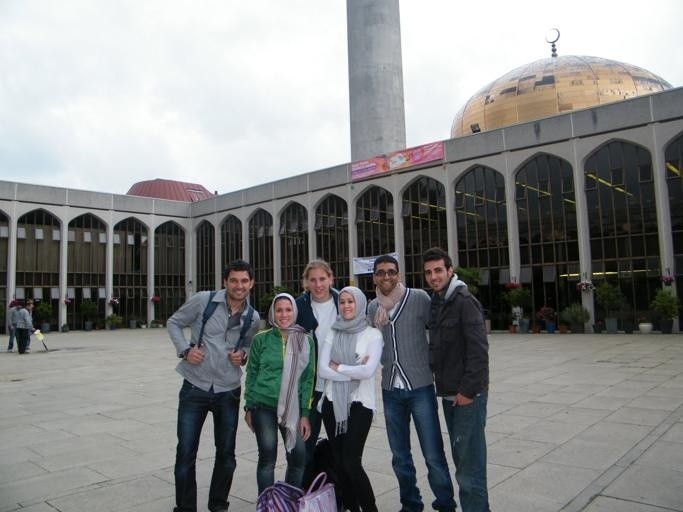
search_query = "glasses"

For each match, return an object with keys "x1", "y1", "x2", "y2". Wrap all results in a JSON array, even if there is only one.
[{"x1": 375, "y1": 269, "x2": 397, "y2": 278}]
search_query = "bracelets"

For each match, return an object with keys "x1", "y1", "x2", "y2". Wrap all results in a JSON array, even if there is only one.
[
  {"x1": 244, "y1": 406, "x2": 255, "y2": 413},
  {"x1": 182, "y1": 349, "x2": 190, "y2": 361}
]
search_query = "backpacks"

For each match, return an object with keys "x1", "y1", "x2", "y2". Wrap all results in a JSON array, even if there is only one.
[{"x1": 311, "y1": 437, "x2": 343, "y2": 510}]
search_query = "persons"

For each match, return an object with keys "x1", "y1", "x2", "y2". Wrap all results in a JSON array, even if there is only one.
[
  {"x1": 6, "y1": 297, "x2": 37, "y2": 354},
  {"x1": 295, "y1": 258, "x2": 341, "y2": 512},
  {"x1": 421, "y1": 246, "x2": 491, "y2": 512},
  {"x1": 244, "y1": 293, "x2": 316, "y2": 497},
  {"x1": 317, "y1": 285, "x2": 384, "y2": 511},
  {"x1": 166, "y1": 259, "x2": 261, "y2": 512},
  {"x1": 366, "y1": 255, "x2": 458, "y2": 512}
]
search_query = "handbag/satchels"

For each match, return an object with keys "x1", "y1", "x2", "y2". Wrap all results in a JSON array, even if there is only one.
[
  {"x1": 256, "y1": 480, "x2": 304, "y2": 511},
  {"x1": 297, "y1": 471, "x2": 338, "y2": 512}
]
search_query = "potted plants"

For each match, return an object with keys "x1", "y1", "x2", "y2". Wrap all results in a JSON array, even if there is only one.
[
  {"x1": 78, "y1": 298, "x2": 98, "y2": 331},
  {"x1": 105, "y1": 313, "x2": 163, "y2": 329},
  {"x1": 501, "y1": 269, "x2": 682, "y2": 335},
  {"x1": 35, "y1": 301, "x2": 53, "y2": 332}
]
